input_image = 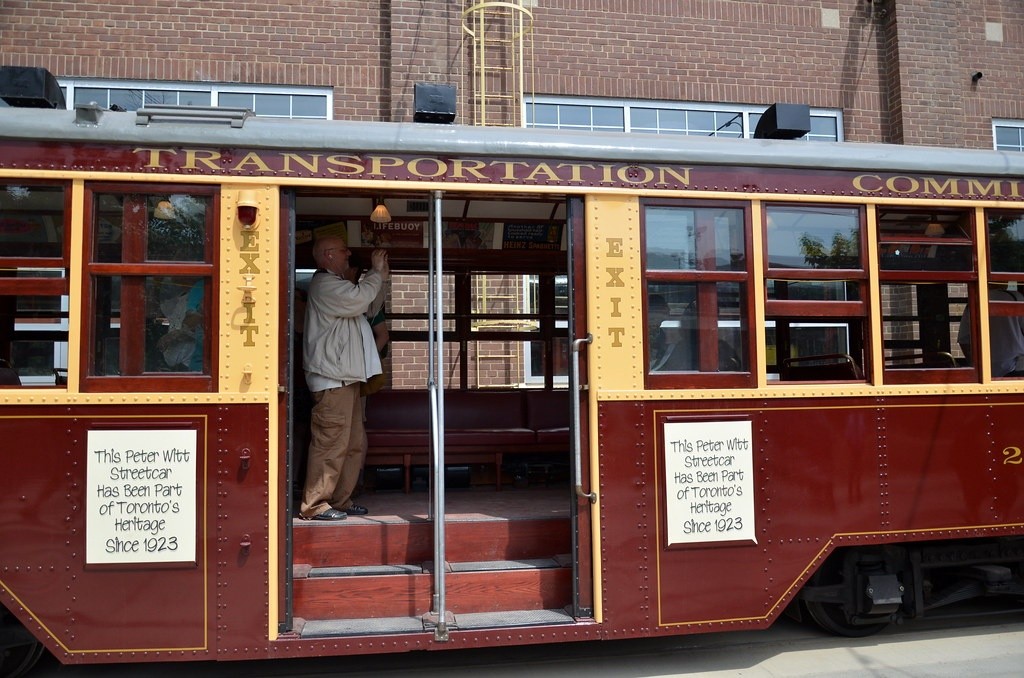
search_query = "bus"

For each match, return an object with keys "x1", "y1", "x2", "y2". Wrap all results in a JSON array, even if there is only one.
[{"x1": 0, "y1": 95, "x2": 1024, "y2": 678}]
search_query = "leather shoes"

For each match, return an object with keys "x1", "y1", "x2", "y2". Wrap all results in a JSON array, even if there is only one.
[
  {"x1": 313, "y1": 508, "x2": 347, "y2": 521},
  {"x1": 345, "y1": 504, "x2": 368, "y2": 515}
]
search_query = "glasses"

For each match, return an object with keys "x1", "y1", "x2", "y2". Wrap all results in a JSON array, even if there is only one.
[{"x1": 325, "y1": 248, "x2": 349, "y2": 256}]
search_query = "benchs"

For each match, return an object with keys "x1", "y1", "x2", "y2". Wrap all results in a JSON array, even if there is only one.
[
  {"x1": 364, "y1": 388, "x2": 570, "y2": 492},
  {"x1": 885, "y1": 352, "x2": 960, "y2": 368},
  {"x1": 782, "y1": 353, "x2": 863, "y2": 380}
]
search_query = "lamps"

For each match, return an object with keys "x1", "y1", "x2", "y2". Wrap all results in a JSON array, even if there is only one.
[
  {"x1": 154, "y1": 196, "x2": 176, "y2": 220},
  {"x1": 370, "y1": 197, "x2": 391, "y2": 223}
]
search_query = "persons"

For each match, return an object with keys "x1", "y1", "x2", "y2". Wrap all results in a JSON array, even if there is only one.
[
  {"x1": 291, "y1": 286, "x2": 314, "y2": 499},
  {"x1": 342, "y1": 255, "x2": 389, "y2": 494},
  {"x1": 296, "y1": 231, "x2": 389, "y2": 521},
  {"x1": 958, "y1": 265, "x2": 1024, "y2": 376},
  {"x1": 652, "y1": 296, "x2": 742, "y2": 371},
  {"x1": 144, "y1": 242, "x2": 205, "y2": 372},
  {"x1": 646, "y1": 294, "x2": 670, "y2": 370}
]
